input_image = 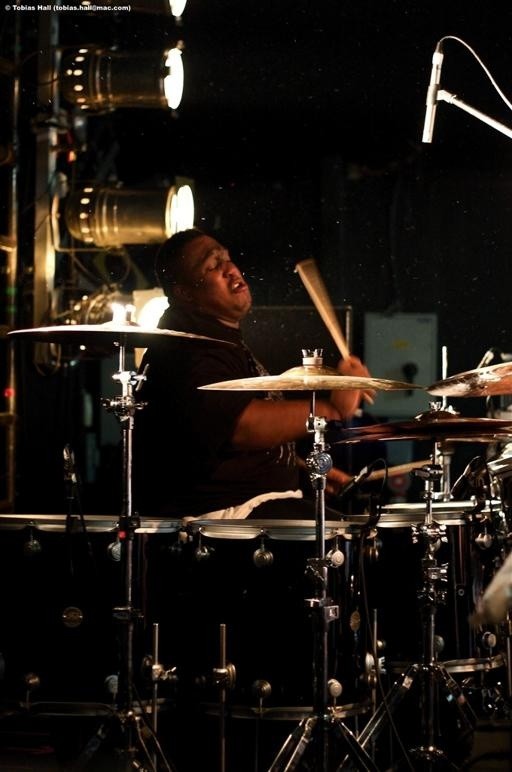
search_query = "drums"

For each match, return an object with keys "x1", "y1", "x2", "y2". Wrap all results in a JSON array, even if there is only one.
[
  {"x1": 381, "y1": 499, "x2": 510, "y2": 771},
  {"x1": 347, "y1": 511, "x2": 477, "y2": 772},
  {"x1": 0, "y1": 513, "x2": 182, "y2": 772},
  {"x1": 186, "y1": 519, "x2": 373, "y2": 772}
]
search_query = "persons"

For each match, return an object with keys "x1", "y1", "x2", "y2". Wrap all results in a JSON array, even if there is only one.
[{"x1": 134, "y1": 229, "x2": 380, "y2": 769}]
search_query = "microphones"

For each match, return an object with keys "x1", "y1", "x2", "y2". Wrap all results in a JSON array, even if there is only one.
[{"x1": 421, "y1": 51, "x2": 443, "y2": 144}]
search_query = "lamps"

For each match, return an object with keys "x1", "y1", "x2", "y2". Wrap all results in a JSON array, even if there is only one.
[{"x1": 0, "y1": 1, "x2": 202, "y2": 378}]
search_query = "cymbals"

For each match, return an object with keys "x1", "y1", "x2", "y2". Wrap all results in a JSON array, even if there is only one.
[
  {"x1": 8, "y1": 317, "x2": 252, "y2": 349},
  {"x1": 338, "y1": 413, "x2": 510, "y2": 443},
  {"x1": 428, "y1": 363, "x2": 512, "y2": 398},
  {"x1": 198, "y1": 350, "x2": 424, "y2": 393}
]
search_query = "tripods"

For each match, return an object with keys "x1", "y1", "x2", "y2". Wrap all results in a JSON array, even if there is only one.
[
  {"x1": 62, "y1": 403, "x2": 178, "y2": 772},
  {"x1": 336, "y1": 552, "x2": 512, "y2": 772},
  {"x1": 268, "y1": 463, "x2": 382, "y2": 772}
]
